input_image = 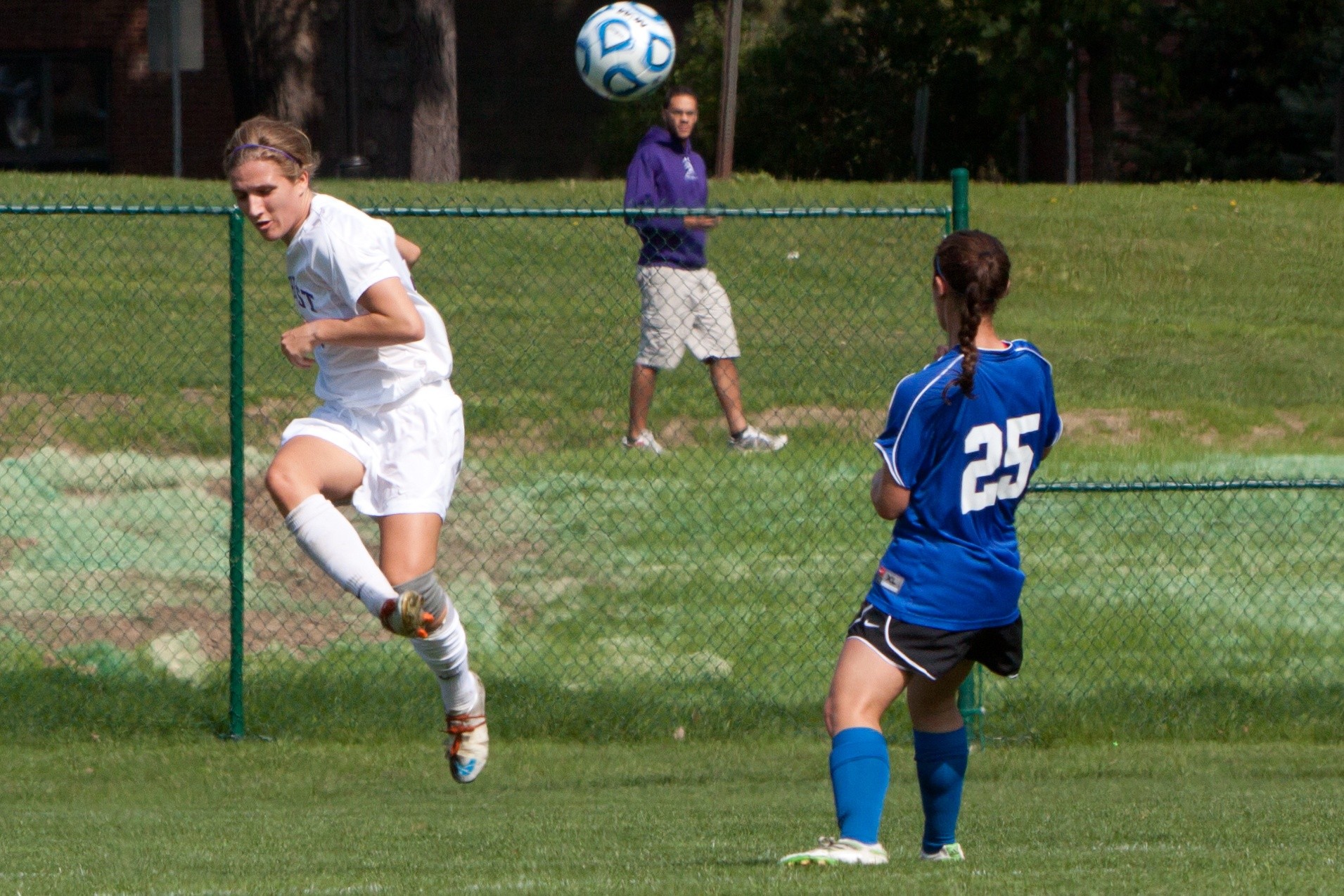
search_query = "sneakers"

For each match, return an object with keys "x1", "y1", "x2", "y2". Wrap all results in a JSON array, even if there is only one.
[
  {"x1": 623, "y1": 432, "x2": 664, "y2": 455},
  {"x1": 779, "y1": 837, "x2": 888, "y2": 867},
  {"x1": 378, "y1": 592, "x2": 432, "y2": 638},
  {"x1": 920, "y1": 843, "x2": 964, "y2": 863},
  {"x1": 438, "y1": 671, "x2": 488, "y2": 784},
  {"x1": 731, "y1": 424, "x2": 789, "y2": 453}
]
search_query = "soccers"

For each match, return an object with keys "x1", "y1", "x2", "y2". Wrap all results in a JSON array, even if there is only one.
[{"x1": 576, "y1": 1, "x2": 675, "y2": 101}]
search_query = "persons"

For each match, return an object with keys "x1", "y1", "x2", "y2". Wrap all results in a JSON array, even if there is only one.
[
  {"x1": 621, "y1": 87, "x2": 788, "y2": 453},
  {"x1": 782, "y1": 232, "x2": 1059, "y2": 864},
  {"x1": 224, "y1": 117, "x2": 491, "y2": 783}
]
